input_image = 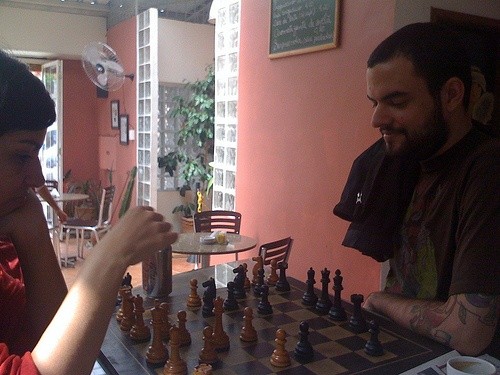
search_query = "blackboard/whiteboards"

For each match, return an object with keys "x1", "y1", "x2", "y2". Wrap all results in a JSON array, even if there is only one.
[{"x1": 268, "y1": 0, "x2": 342, "y2": 59}]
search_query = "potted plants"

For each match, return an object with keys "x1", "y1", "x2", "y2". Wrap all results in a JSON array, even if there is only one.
[
  {"x1": 157, "y1": 57, "x2": 216, "y2": 233},
  {"x1": 67, "y1": 177, "x2": 100, "y2": 220}
]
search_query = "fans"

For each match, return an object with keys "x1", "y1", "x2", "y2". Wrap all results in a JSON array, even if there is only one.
[{"x1": 82, "y1": 42, "x2": 135, "y2": 92}]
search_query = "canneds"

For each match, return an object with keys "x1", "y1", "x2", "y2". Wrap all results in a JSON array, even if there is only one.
[{"x1": 141, "y1": 245, "x2": 172, "y2": 300}]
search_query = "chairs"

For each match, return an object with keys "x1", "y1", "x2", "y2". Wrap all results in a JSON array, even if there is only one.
[
  {"x1": 259, "y1": 234, "x2": 295, "y2": 270},
  {"x1": 194, "y1": 210, "x2": 241, "y2": 270},
  {"x1": 63, "y1": 185, "x2": 116, "y2": 267}
]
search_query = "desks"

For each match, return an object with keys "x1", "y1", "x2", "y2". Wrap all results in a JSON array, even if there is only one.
[
  {"x1": 35, "y1": 192, "x2": 89, "y2": 268},
  {"x1": 89, "y1": 256, "x2": 500, "y2": 375},
  {"x1": 171, "y1": 232, "x2": 257, "y2": 269}
]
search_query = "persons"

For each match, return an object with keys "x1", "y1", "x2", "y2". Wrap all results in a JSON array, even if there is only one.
[
  {"x1": 365, "y1": 22, "x2": 500, "y2": 356},
  {"x1": 0, "y1": 50, "x2": 179, "y2": 375}
]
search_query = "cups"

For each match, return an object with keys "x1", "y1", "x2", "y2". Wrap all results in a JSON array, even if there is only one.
[{"x1": 447, "y1": 356, "x2": 496, "y2": 375}]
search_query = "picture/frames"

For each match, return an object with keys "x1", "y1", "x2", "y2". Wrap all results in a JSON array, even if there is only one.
[
  {"x1": 120, "y1": 115, "x2": 129, "y2": 144},
  {"x1": 111, "y1": 100, "x2": 119, "y2": 129}
]
